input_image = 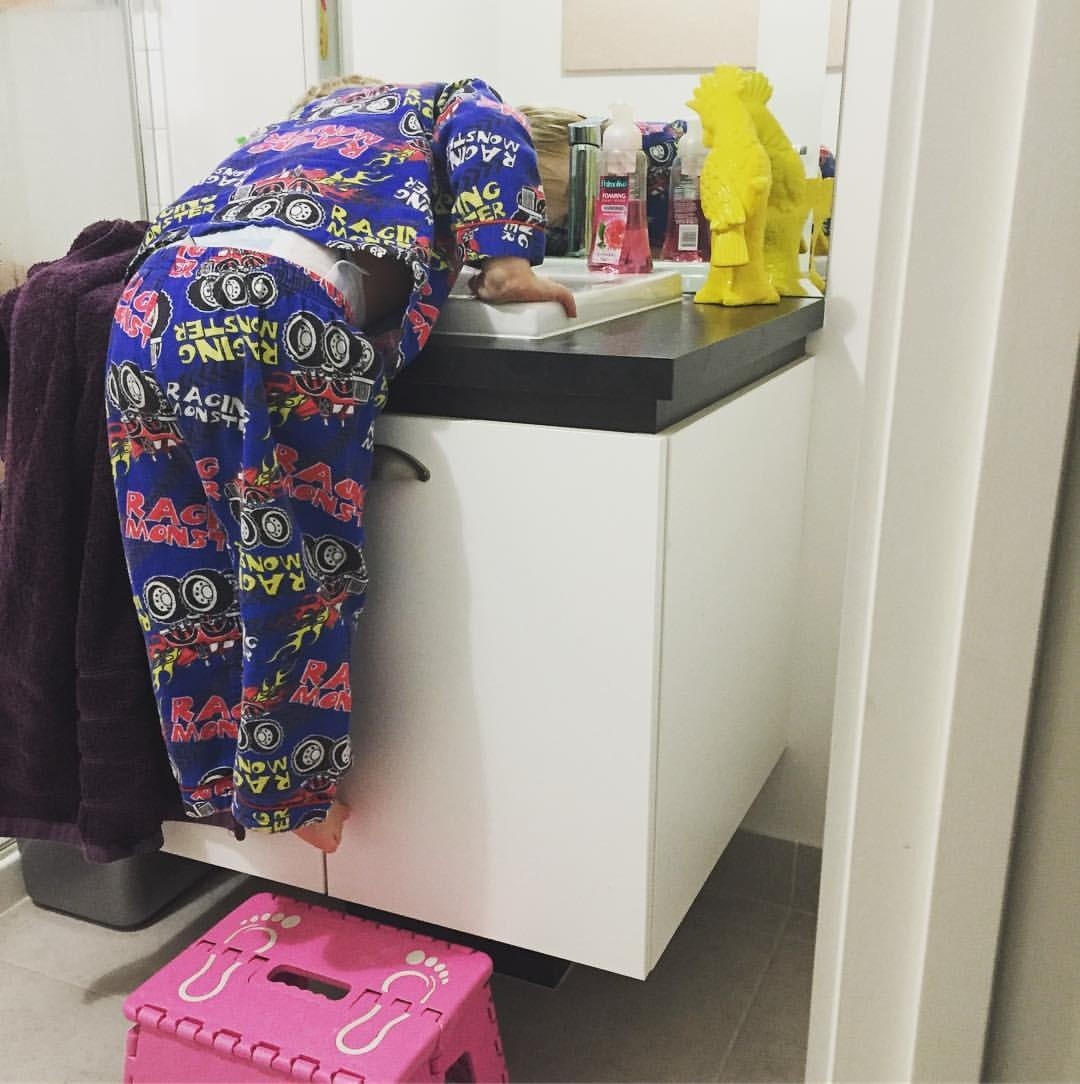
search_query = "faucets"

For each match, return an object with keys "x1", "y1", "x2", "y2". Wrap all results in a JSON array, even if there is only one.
[{"x1": 568, "y1": 116, "x2": 609, "y2": 255}]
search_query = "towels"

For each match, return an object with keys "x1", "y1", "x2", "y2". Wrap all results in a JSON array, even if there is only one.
[{"x1": 0, "y1": 213, "x2": 163, "y2": 868}]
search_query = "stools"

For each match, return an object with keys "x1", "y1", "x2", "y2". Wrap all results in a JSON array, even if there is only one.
[{"x1": 124, "y1": 892, "x2": 510, "y2": 1084}]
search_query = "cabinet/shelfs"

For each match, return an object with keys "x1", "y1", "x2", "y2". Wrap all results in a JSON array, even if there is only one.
[{"x1": 162, "y1": 337, "x2": 816, "y2": 980}]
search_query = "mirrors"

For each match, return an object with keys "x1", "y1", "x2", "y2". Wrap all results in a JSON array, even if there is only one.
[{"x1": 338, "y1": 0, "x2": 852, "y2": 299}]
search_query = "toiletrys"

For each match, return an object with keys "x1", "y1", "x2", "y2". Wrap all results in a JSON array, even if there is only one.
[
  {"x1": 659, "y1": 113, "x2": 715, "y2": 264},
  {"x1": 588, "y1": 99, "x2": 652, "y2": 273}
]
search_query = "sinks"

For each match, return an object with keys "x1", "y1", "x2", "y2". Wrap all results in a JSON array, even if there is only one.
[{"x1": 427, "y1": 263, "x2": 682, "y2": 342}]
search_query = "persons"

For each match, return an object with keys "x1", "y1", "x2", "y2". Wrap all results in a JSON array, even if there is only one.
[{"x1": 107, "y1": 77, "x2": 576, "y2": 852}]
